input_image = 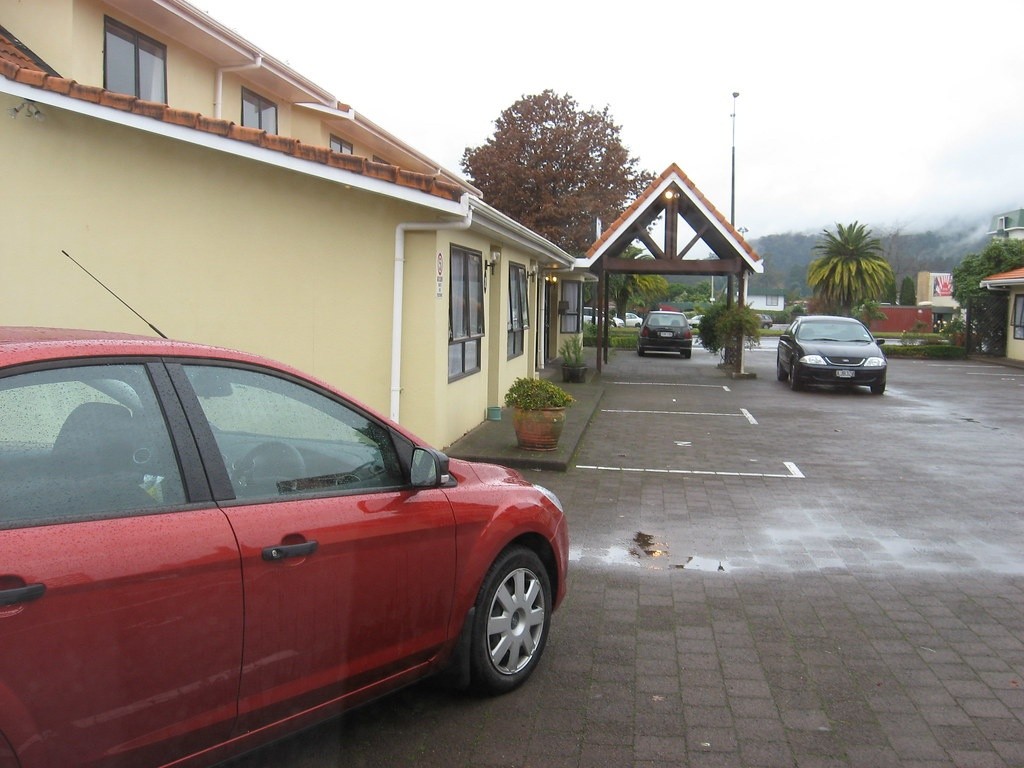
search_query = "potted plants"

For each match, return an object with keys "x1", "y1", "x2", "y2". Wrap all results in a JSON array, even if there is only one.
[
  {"x1": 558, "y1": 328, "x2": 590, "y2": 383},
  {"x1": 505, "y1": 376, "x2": 577, "y2": 451}
]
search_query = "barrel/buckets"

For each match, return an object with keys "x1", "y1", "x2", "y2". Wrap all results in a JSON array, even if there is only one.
[{"x1": 487, "y1": 407, "x2": 501, "y2": 421}]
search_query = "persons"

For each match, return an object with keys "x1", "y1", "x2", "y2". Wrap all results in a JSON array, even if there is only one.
[{"x1": 933, "y1": 318, "x2": 947, "y2": 333}]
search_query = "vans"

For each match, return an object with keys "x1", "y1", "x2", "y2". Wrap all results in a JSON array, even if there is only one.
[{"x1": 583, "y1": 305, "x2": 625, "y2": 328}]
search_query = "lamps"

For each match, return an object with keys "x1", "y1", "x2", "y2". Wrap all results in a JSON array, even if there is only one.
[
  {"x1": 484, "y1": 251, "x2": 500, "y2": 294},
  {"x1": 527, "y1": 265, "x2": 538, "y2": 283}
]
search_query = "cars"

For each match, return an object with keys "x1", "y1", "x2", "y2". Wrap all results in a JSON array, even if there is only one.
[
  {"x1": 1, "y1": 316, "x2": 571, "y2": 767},
  {"x1": 625, "y1": 313, "x2": 643, "y2": 327},
  {"x1": 688, "y1": 314, "x2": 705, "y2": 328},
  {"x1": 634, "y1": 310, "x2": 693, "y2": 359},
  {"x1": 757, "y1": 314, "x2": 773, "y2": 329},
  {"x1": 775, "y1": 315, "x2": 889, "y2": 395}
]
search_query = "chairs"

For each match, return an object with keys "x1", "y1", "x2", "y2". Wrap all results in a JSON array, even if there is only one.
[
  {"x1": 800, "y1": 327, "x2": 815, "y2": 337},
  {"x1": 119, "y1": 408, "x2": 188, "y2": 508},
  {"x1": 650, "y1": 317, "x2": 659, "y2": 325},
  {"x1": 46, "y1": 402, "x2": 131, "y2": 482},
  {"x1": 671, "y1": 320, "x2": 680, "y2": 326}
]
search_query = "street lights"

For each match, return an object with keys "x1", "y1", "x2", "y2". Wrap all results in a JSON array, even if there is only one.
[{"x1": 730, "y1": 91, "x2": 739, "y2": 227}]
